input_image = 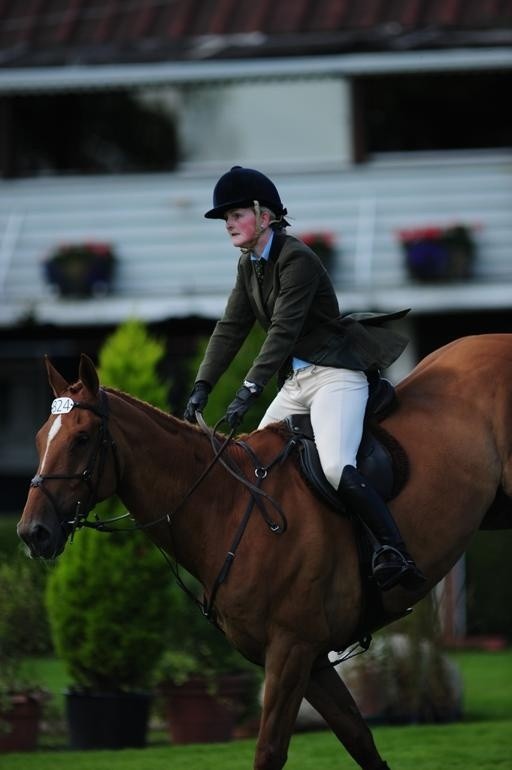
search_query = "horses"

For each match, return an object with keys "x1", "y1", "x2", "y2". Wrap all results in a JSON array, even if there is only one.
[{"x1": 11, "y1": 328, "x2": 512, "y2": 769}]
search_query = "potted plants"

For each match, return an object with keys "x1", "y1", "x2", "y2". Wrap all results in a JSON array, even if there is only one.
[{"x1": 1, "y1": 323, "x2": 269, "y2": 755}]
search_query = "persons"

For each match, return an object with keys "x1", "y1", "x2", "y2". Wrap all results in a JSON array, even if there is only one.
[{"x1": 181, "y1": 166, "x2": 420, "y2": 595}]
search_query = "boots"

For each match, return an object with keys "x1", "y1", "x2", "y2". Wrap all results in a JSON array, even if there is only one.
[{"x1": 337, "y1": 464, "x2": 427, "y2": 591}]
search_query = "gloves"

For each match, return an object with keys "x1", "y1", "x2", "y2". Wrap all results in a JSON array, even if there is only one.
[
  {"x1": 184, "y1": 382, "x2": 210, "y2": 421},
  {"x1": 227, "y1": 385, "x2": 258, "y2": 429}
]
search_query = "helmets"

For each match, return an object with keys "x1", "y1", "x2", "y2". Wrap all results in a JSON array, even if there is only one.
[{"x1": 204, "y1": 165, "x2": 284, "y2": 218}]
